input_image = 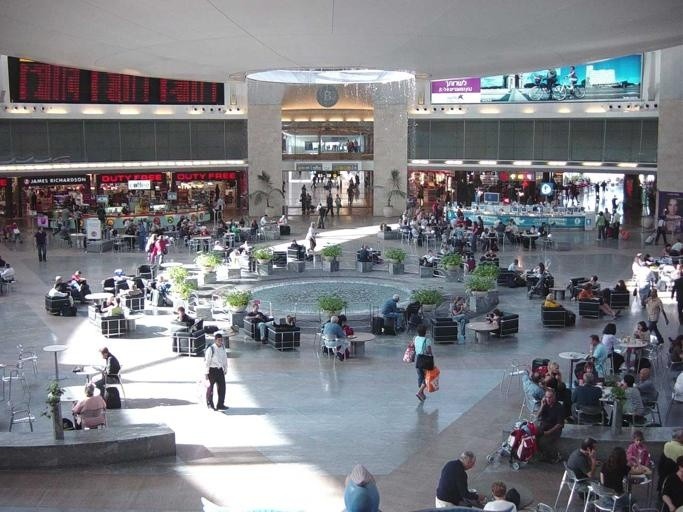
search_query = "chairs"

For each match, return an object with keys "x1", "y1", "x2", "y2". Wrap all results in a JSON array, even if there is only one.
[
  {"x1": 498, "y1": 333, "x2": 683, "y2": 512},
  {"x1": 313, "y1": 326, "x2": 376, "y2": 361},
  {"x1": 101, "y1": 364, "x2": 126, "y2": 407},
  {"x1": 355, "y1": 248, "x2": 381, "y2": 263},
  {"x1": 2, "y1": 274, "x2": 17, "y2": 297},
  {"x1": 44, "y1": 221, "x2": 307, "y2": 357},
  {"x1": 539, "y1": 255, "x2": 681, "y2": 327},
  {"x1": 0, "y1": 343, "x2": 40, "y2": 432},
  {"x1": 379, "y1": 221, "x2": 552, "y2": 342},
  {"x1": 71, "y1": 407, "x2": 107, "y2": 431}
]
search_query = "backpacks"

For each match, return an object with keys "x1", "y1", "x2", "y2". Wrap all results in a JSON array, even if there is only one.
[{"x1": 104, "y1": 387, "x2": 121, "y2": 409}]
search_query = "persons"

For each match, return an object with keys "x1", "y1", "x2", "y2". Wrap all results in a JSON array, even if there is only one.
[
  {"x1": 542, "y1": 68, "x2": 560, "y2": 99},
  {"x1": 568, "y1": 65, "x2": 579, "y2": 99}
]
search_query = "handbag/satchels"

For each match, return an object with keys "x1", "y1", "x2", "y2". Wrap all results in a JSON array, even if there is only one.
[
  {"x1": 424, "y1": 367, "x2": 439, "y2": 393},
  {"x1": 401, "y1": 346, "x2": 415, "y2": 363},
  {"x1": 516, "y1": 436, "x2": 539, "y2": 463},
  {"x1": 415, "y1": 353, "x2": 433, "y2": 370}
]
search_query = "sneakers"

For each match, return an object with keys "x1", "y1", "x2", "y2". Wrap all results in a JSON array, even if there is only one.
[
  {"x1": 415, "y1": 391, "x2": 426, "y2": 401},
  {"x1": 207, "y1": 404, "x2": 216, "y2": 411},
  {"x1": 216, "y1": 406, "x2": 228, "y2": 410},
  {"x1": 335, "y1": 352, "x2": 343, "y2": 361},
  {"x1": 656, "y1": 341, "x2": 665, "y2": 345}
]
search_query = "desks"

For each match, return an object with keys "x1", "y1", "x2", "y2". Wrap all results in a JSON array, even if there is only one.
[
  {"x1": 75, "y1": 367, "x2": 101, "y2": 386},
  {"x1": 48, "y1": 385, "x2": 100, "y2": 406},
  {"x1": 42, "y1": 345, "x2": 68, "y2": 381}
]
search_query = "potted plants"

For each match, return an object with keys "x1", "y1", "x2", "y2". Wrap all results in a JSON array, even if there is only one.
[
  {"x1": 369, "y1": 168, "x2": 406, "y2": 218},
  {"x1": 319, "y1": 241, "x2": 344, "y2": 262},
  {"x1": 249, "y1": 170, "x2": 286, "y2": 217},
  {"x1": 316, "y1": 292, "x2": 347, "y2": 326}
]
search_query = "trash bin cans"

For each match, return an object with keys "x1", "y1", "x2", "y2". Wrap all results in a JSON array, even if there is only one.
[{"x1": 70, "y1": 233, "x2": 86, "y2": 252}]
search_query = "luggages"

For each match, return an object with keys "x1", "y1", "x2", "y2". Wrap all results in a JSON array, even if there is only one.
[{"x1": 371, "y1": 305, "x2": 384, "y2": 336}]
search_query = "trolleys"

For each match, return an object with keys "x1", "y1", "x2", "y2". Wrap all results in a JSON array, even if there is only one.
[{"x1": 485, "y1": 421, "x2": 540, "y2": 471}]
